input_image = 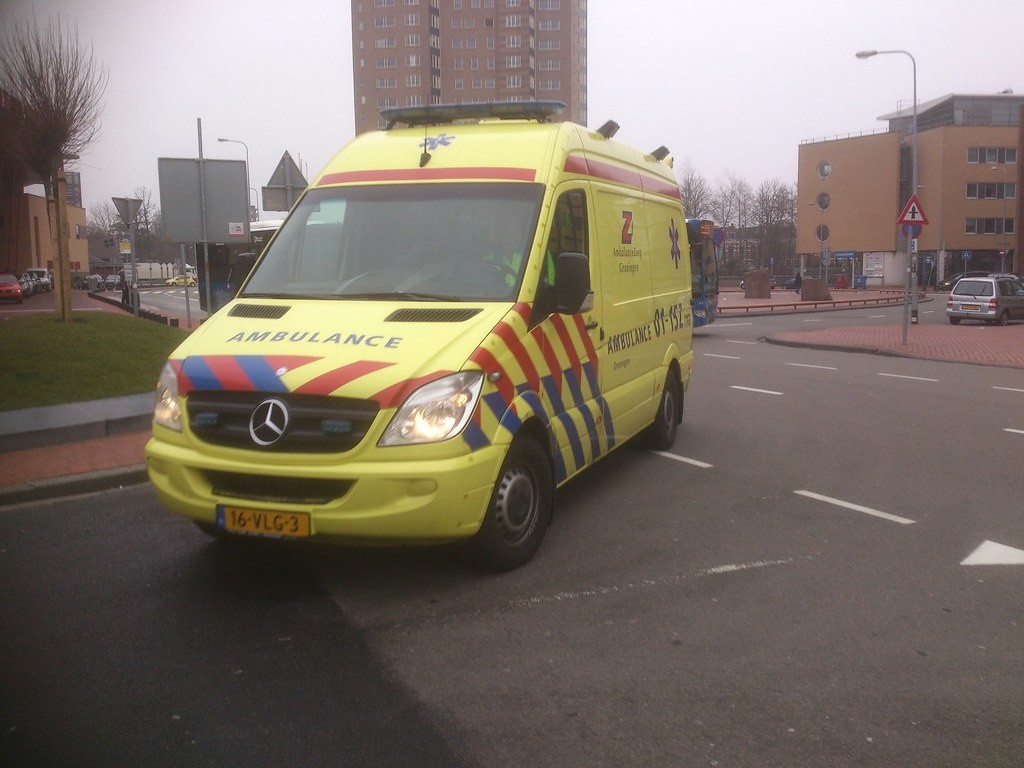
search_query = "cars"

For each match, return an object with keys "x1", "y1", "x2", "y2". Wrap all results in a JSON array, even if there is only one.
[
  {"x1": 105, "y1": 275, "x2": 121, "y2": 286},
  {"x1": 0, "y1": 270, "x2": 37, "y2": 305},
  {"x1": 165, "y1": 274, "x2": 197, "y2": 286},
  {"x1": 84, "y1": 275, "x2": 105, "y2": 289}
]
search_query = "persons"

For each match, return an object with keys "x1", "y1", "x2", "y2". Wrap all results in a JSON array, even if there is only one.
[
  {"x1": 477, "y1": 201, "x2": 557, "y2": 298},
  {"x1": 794, "y1": 273, "x2": 802, "y2": 295}
]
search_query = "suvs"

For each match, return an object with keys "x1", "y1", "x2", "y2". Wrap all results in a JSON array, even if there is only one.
[
  {"x1": 946, "y1": 273, "x2": 1024, "y2": 326},
  {"x1": 27, "y1": 268, "x2": 52, "y2": 290}
]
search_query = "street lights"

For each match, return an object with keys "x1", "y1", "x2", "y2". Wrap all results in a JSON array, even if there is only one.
[
  {"x1": 217, "y1": 139, "x2": 251, "y2": 244},
  {"x1": 856, "y1": 50, "x2": 919, "y2": 344}
]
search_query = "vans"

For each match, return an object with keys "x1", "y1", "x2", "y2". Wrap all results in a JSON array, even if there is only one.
[{"x1": 939, "y1": 270, "x2": 992, "y2": 291}]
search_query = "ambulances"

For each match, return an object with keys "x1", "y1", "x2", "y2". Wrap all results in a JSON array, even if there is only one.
[{"x1": 142, "y1": 99, "x2": 694, "y2": 571}]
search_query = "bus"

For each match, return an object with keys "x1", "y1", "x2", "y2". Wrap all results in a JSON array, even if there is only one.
[{"x1": 197, "y1": 218, "x2": 721, "y2": 330}]
